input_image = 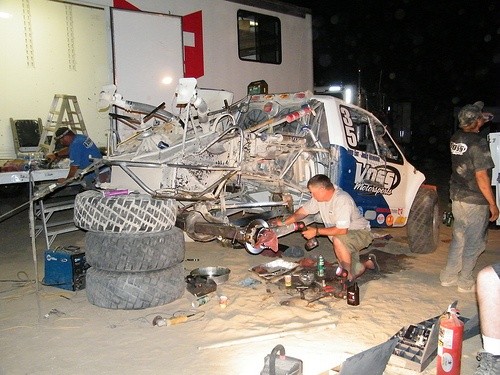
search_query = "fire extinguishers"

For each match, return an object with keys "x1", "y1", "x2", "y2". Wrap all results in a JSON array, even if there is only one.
[{"x1": 436, "y1": 300, "x2": 464, "y2": 375}]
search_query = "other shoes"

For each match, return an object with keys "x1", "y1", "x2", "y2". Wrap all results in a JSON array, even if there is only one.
[{"x1": 474, "y1": 352, "x2": 500, "y2": 375}]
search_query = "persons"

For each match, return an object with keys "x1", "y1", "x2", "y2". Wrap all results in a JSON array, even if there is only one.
[
  {"x1": 441, "y1": 101, "x2": 500, "y2": 293},
  {"x1": 47, "y1": 127, "x2": 102, "y2": 190},
  {"x1": 475, "y1": 261, "x2": 500, "y2": 375},
  {"x1": 272, "y1": 175, "x2": 380, "y2": 282}
]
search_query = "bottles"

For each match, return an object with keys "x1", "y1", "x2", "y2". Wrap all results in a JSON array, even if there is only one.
[
  {"x1": 317, "y1": 254, "x2": 326, "y2": 279},
  {"x1": 191, "y1": 292, "x2": 216, "y2": 308}
]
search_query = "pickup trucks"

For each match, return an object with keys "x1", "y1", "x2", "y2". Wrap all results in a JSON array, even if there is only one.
[{"x1": 96, "y1": 89, "x2": 442, "y2": 254}]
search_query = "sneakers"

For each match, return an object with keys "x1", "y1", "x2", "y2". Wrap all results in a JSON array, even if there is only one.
[
  {"x1": 457, "y1": 281, "x2": 478, "y2": 293},
  {"x1": 338, "y1": 280, "x2": 354, "y2": 290},
  {"x1": 368, "y1": 254, "x2": 381, "y2": 273},
  {"x1": 440, "y1": 276, "x2": 458, "y2": 286}
]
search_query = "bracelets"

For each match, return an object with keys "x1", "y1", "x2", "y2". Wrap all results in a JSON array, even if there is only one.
[{"x1": 282, "y1": 219, "x2": 285, "y2": 225}]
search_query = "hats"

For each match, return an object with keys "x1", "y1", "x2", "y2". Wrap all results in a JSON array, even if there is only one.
[{"x1": 458, "y1": 101, "x2": 485, "y2": 128}]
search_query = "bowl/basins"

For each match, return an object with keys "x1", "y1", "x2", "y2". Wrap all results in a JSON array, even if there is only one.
[{"x1": 190, "y1": 266, "x2": 231, "y2": 283}]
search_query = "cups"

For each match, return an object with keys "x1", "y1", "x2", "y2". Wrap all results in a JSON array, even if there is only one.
[{"x1": 218, "y1": 296, "x2": 227, "y2": 309}]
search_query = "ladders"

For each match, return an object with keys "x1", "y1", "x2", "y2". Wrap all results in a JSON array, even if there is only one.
[{"x1": 31, "y1": 95, "x2": 104, "y2": 251}]
